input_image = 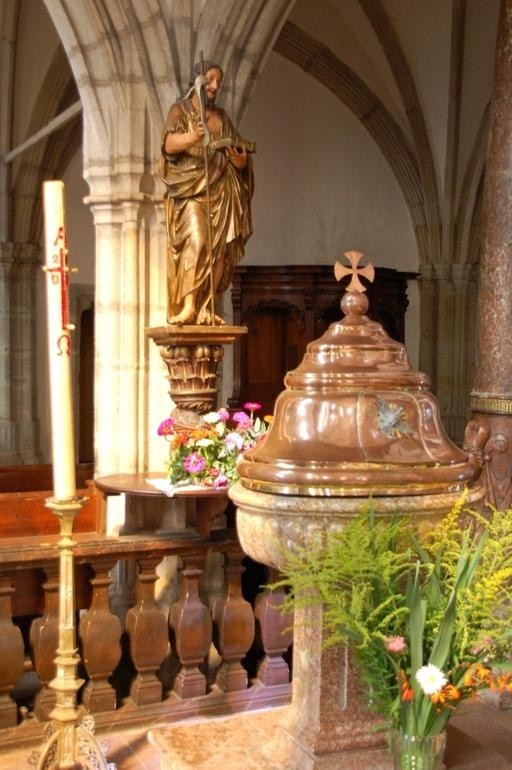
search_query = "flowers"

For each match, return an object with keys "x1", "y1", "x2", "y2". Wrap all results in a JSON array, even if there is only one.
[
  {"x1": 157, "y1": 398, "x2": 275, "y2": 490},
  {"x1": 256, "y1": 488, "x2": 512, "y2": 770}
]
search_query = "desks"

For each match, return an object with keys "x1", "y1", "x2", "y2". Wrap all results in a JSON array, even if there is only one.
[{"x1": 95, "y1": 470, "x2": 232, "y2": 617}]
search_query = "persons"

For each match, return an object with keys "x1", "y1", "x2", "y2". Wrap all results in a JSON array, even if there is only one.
[{"x1": 161, "y1": 61, "x2": 255, "y2": 325}]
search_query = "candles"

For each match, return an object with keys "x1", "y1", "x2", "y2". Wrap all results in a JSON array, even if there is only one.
[{"x1": 40, "y1": 179, "x2": 78, "y2": 502}]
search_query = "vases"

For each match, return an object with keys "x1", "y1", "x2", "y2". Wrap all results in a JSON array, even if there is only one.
[{"x1": 396, "y1": 728, "x2": 448, "y2": 770}]
